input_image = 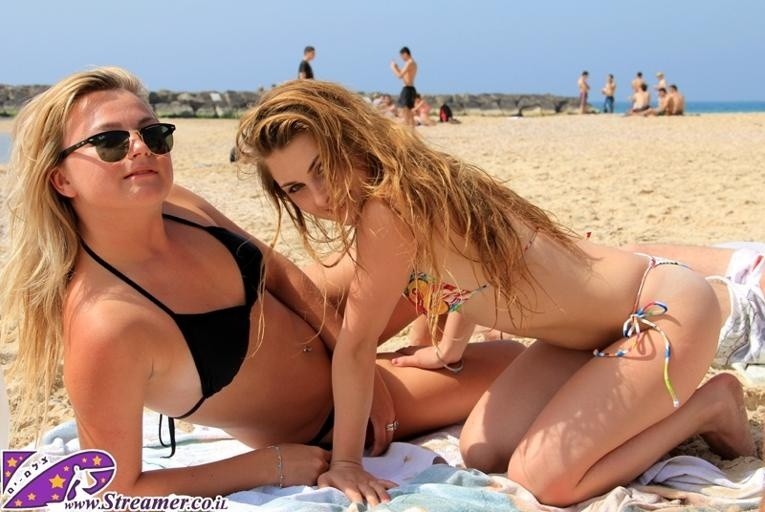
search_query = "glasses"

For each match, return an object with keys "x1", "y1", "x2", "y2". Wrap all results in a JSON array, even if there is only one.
[{"x1": 54, "y1": 121, "x2": 176, "y2": 164}]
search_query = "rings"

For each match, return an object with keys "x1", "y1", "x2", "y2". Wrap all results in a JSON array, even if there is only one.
[{"x1": 385, "y1": 420, "x2": 400, "y2": 432}]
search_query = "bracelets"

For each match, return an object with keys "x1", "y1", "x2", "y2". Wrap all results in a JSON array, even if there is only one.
[
  {"x1": 268, "y1": 446, "x2": 285, "y2": 489},
  {"x1": 436, "y1": 343, "x2": 465, "y2": 373}
]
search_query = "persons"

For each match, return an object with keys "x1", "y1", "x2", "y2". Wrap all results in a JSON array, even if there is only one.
[
  {"x1": 299, "y1": 47, "x2": 315, "y2": 79},
  {"x1": 601, "y1": 75, "x2": 615, "y2": 114},
  {"x1": 366, "y1": 92, "x2": 462, "y2": 126},
  {"x1": 622, "y1": 240, "x2": 765, "y2": 370},
  {"x1": 237, "y1": 78, "x2": 759, "y2": 505},
  {"x1": 1, "y1": 65, "x2": 527, "y2": 496},
  {"x1": 632, "y1": 72, "x2": 684, "y2": 116},
  {"x1": 388, "y1": 46, "x2": 417, "y2": 127},
  {"x1": 579, "y1": 71, "x2": 590, "y2": 113}
]
